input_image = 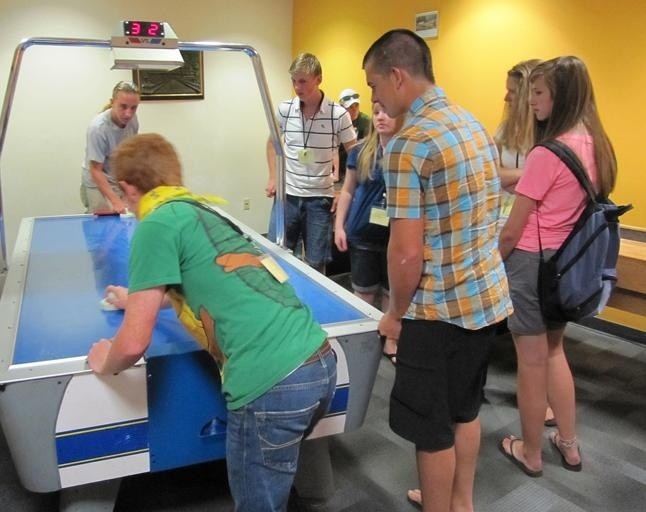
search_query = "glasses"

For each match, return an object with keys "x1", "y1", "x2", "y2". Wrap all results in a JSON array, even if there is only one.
[{"x1": 343, "y1": 94, "x2": 359, "y2": 101}]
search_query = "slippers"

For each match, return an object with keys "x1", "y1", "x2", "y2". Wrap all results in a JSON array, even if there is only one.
[
  {"x1": 548, "y1": 429, "x2": 583, "y2": 471},
  {"x1": 500, "y1": 433, "x2": 544, "y2": 477}
]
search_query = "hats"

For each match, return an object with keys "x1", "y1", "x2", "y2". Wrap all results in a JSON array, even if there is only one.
[{"x1": 339, "y1": 88, "x2": 360, "y2": 108}]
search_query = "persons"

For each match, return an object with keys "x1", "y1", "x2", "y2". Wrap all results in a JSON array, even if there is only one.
[
  {"x1": 357, "y1": 26, "x2": 517, "y2": 511},
  {"x1": 264, "y1": 52, "x2": 356, "y2": 272},
  {"x1": 78, "y1": 78, "x2": 141, "y2": 217},
  {"x1": 331, "y1": 87, "x2": 377, "y2": 185},
  {"x1": 488, "y1": 57, "x2": 574, "y2": 431},
  {"x1": 495, "y1": 55, "x2": 619, "y2": 479},
  {"x1": 82, "y1": 129, "x2": 339, "y2": 510},
  {"x1": 334, "y1": 97, "x2": 405, "y2": 371}
]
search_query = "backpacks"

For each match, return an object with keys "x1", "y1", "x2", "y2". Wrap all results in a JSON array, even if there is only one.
[{"x1": 537, "y1": 194, "x2": 634, "y2": 324}]
